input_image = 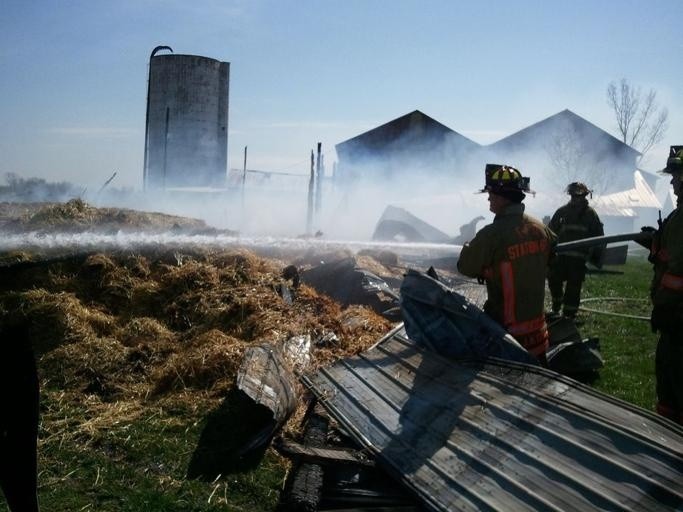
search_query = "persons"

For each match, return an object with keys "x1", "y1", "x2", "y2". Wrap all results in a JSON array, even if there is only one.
[
  {"x1": 458, "y1": 164, "x2": 558, "y2": 368},
  {"x1": 546, "y1": 180, "x2": 607, "y2": 319},
  {"x1": 649, "y1": 145, "x2": 683, "y2": 426}
]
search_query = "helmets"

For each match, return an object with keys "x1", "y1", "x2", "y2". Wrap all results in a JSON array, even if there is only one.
[
  {"x1": 478, "y1": 164, "x2": 529, "y2": 192},
  {"x1": 568, "y1": 182, "x2": 588, "y2": 197},
  {"x1": 663, "y1": 146, "x2": 683, "y2": 172}
]
